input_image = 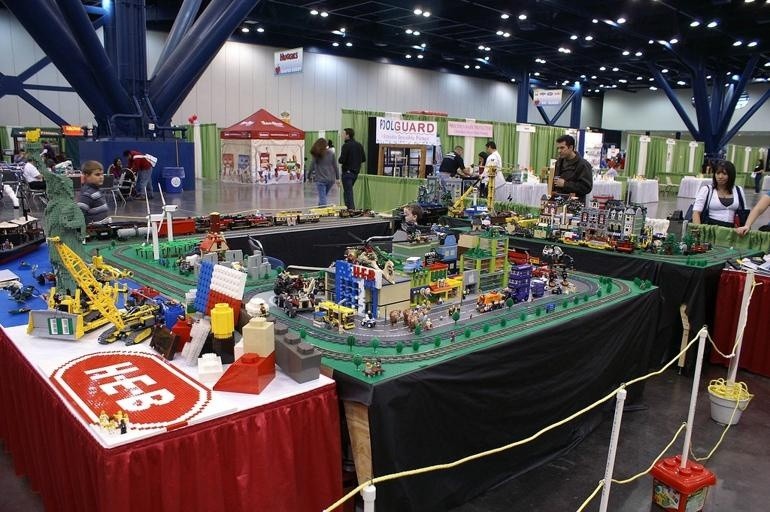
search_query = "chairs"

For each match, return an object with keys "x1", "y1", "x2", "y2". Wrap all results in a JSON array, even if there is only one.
[{"x1": 0, "y1": 160, "x2": 137, "y2": 210}]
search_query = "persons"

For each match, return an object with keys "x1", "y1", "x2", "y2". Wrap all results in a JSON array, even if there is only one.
[
  {"x1": 21, "y1": 156, "x2": 46, "y2": 190},
  {"x1": 338, "y1": 127, "x2": 368, "y2": 209},
  {"x1": 77, "y1": 160, "x2": 109, "y2": 224},
  {"x1": 306, "y1": 137, "x2": 340, "y2": 207},
  {"x1": 690, "y1": 159, "x2": 747, "y2": 229},
  {"x1": 123, "y1": 149, "x2": 156, "y2": 201},
  {"x1": 735, "y1": 190, "x2": 770, "y2": 238},
  {"x1": 440, "y1": 145, "x2": 473, "y2": 179},
  {"x1": 14, "y1": 149, "x2": 29, "y2": 163},
  {"x1": 45, "y1": 142, "x2": 56, "y2": 158},
  {"x1": 403, "y1": 205, "x2": 423, "y2": 225},
  {"x1": 111, "y1": 158, "x2": 123, "y2": 179},
  {"x1": 753, "y1": 159, "x2": 764, "y2": 195},
  {"x1": 479, "y1": 140, "x2": 506, "y2": 190},
  {"x1": 477, "y1": 152, "x2": 488, "y2": 175},
  {"x1": 42, "y1": 153, "x2": 57, "y2": 169},
  {"x1": 327, "y1": 139, "x2": 336, "y2": 157},
  {"x1": 550, "y1": 133, "x2": 593, "y2": 207}
]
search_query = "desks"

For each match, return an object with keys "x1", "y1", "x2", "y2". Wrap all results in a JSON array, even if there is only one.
[
  {"x1": 495, "y1": 175, "x2": 713, "y2": 209},
  {"x1": 1, "y1": 241, "x2": 339, "y2": 512}
]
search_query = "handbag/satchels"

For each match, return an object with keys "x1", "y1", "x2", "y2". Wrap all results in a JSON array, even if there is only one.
[
  {"x1": 751, "y1": 172, "x2": 757, "y2": 178},
  {"x1": 734, "y1": 209, "x2": 750, "y2": 228},
  {"x1": 144, "y1": 153, "x2": 158, "y2": 168},
  {"x1": 685, "y1": 203, "x2": 710, "y2": 224}
]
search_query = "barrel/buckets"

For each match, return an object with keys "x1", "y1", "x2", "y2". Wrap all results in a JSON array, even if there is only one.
[{"x1": 708, "y1": 387, "x2": 749, "y2": 425}]
